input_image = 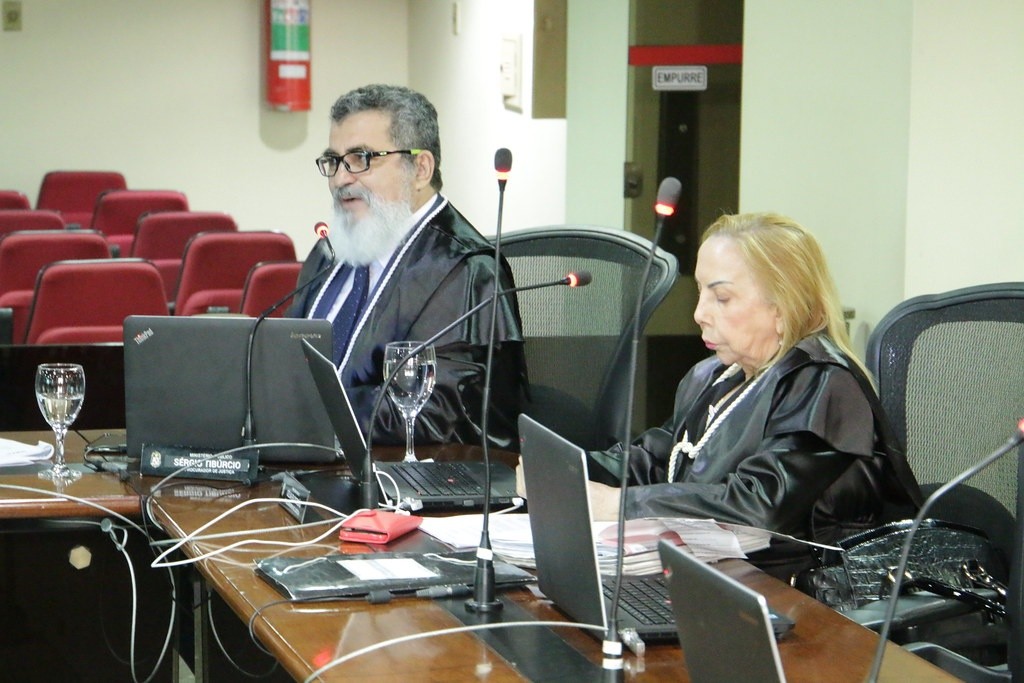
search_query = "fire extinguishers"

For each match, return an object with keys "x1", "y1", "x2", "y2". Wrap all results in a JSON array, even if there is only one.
[{"x1": 264, "y1": 0, "x2": 312, "y2": 110}]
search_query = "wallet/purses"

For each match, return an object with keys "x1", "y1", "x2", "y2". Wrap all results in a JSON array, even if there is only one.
[{"x1": 339, "y1": 510, "x2": 423, "y2": 544}]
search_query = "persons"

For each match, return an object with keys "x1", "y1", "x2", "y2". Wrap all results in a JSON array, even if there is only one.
[
  {"x1": 281, "y1": 83, "x2": 531, "y2": 447},
  {"x1": 515, "y1": 215, "x2": 925, "y2": 601}
]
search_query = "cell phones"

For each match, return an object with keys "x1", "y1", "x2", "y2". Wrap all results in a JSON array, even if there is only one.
[{"x1": 86, "y1": 433, "x2": 126, "y2": 453}]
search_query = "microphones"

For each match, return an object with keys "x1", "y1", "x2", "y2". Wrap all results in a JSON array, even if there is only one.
[
  {"x1": 241, "y1": 223, "x2": 335, "y2": 449},
  {"x1": 364, "y1": 272, "x2": 592, "y2": 509},
  {"x1": 600, "y1": 178, "x2": 679, "y2": 683},
  {"x1": 464, "y1": 148, "x2": 512, "y2": 613},
  {"x1": 867, "y1": 418, "x2": 1024, "y2": 683}
]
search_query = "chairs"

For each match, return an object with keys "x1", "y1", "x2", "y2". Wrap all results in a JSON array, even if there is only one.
[
  {"x1": 0, "y1": 169, "x2": 310, "y2": 345},
  {"x1": 864, "y1": 280, "x2": 1024, "y2": 521},
  {"x1": 484, "y1": 225, "x2": 678, "y2": 483}
]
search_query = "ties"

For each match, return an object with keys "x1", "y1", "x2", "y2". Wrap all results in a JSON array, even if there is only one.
[{"x1": 329, "y1": 264, "x2": 371, "y2": 370}]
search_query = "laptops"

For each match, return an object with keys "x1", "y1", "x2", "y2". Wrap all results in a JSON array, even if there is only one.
[
  {"x1": 519, "y1": 412, "x2": 678, "y2": 643},
  {"x1": 659, "y1": 539, "x2": 787, "y2": 683},
  {"x1": 301, "y1": 339, "x2": 526, "y2": 510},
  {"x1": 124, "y1": 315, "x2": 345, "y2": 463}
]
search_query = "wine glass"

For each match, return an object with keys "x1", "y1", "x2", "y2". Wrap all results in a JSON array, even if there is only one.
[
  {"x1": 382, "y1": 341, "x2": 437, "y2": 463},
  {"x1": 35, "y1": 363, "x2": 85, "y2": 482}
]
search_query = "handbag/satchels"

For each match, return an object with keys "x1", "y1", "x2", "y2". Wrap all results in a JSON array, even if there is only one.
[{"x1": 789, "y1": 520, "x2": 1006, "y2": 617}]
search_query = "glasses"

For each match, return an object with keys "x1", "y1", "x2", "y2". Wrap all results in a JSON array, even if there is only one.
[{"x1": 316, "y1": 149, "x2": 421, "y2": 177}]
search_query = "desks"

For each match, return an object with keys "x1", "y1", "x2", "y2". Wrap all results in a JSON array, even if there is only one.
[{"x1": 1, "y1": 429, "x2": 966, "y2": 683}]
